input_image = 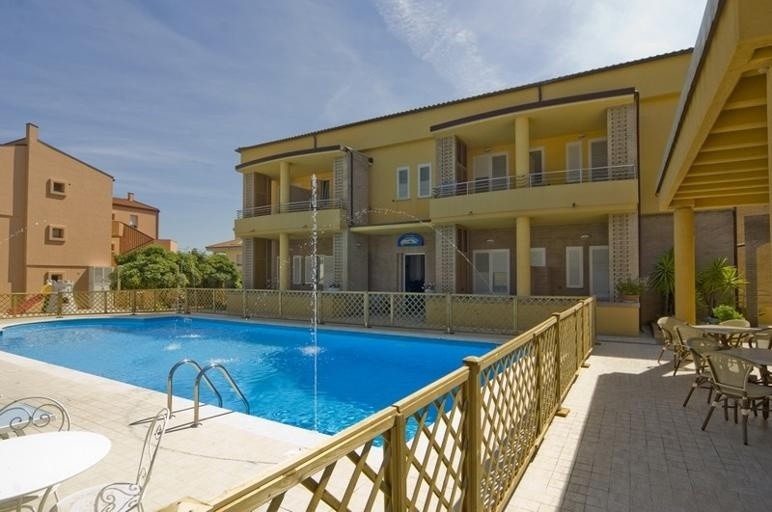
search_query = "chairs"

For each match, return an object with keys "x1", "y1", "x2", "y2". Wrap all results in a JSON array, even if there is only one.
[
  {"x1": 656, "y1": 316, "x2": 772, "y2": 445},
  {"x1": 0, "y1": 396, "x2": 170, "y2": 511}
]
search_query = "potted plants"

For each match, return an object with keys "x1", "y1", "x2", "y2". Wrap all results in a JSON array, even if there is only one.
[
  {"x1": 648, "y1": 245, "x2": 673, "y2": 341},
  {"x1": 614, "y1": 276, "x2": 647, "y2": 302}
]
